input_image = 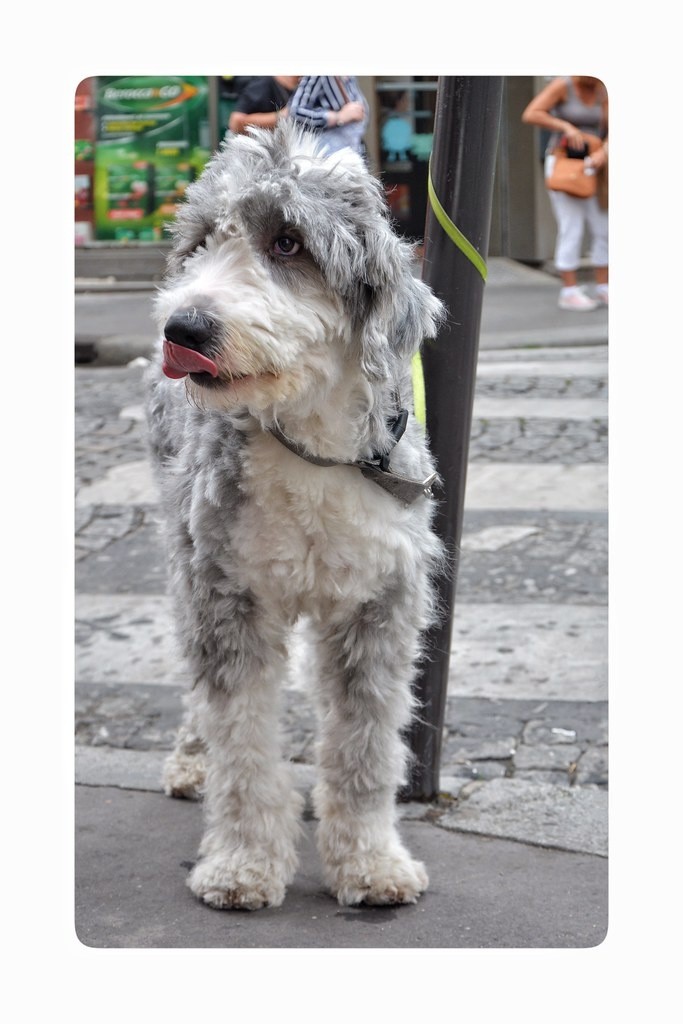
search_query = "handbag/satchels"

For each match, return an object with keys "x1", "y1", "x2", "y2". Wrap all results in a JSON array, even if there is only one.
[{"x1": 547, "y1": 133, "x2": 601, "y2": 197}]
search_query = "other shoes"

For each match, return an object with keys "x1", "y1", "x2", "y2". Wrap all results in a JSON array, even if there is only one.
[
  {"x1": 558, "y1": 288, "x2": 598, "y2": 312},
  {"x1": 597, "y1": 289, "x2": 608, "y2": 304}
]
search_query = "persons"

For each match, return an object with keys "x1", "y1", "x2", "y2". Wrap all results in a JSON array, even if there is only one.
[
  {"x1": 229, "y1": 76, "x2": 369, "y2": 149},
  {"x1": 377, "y1": 90, "x2": 417, "y2": 161},
  {"x1": 521, "y1": 76, "x2": 610, "y2": 311}
]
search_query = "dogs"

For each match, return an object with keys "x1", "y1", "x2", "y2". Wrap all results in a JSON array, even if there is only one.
[{"x1": 148, "y1": 114, "x2": 447, "y2": 913}]
